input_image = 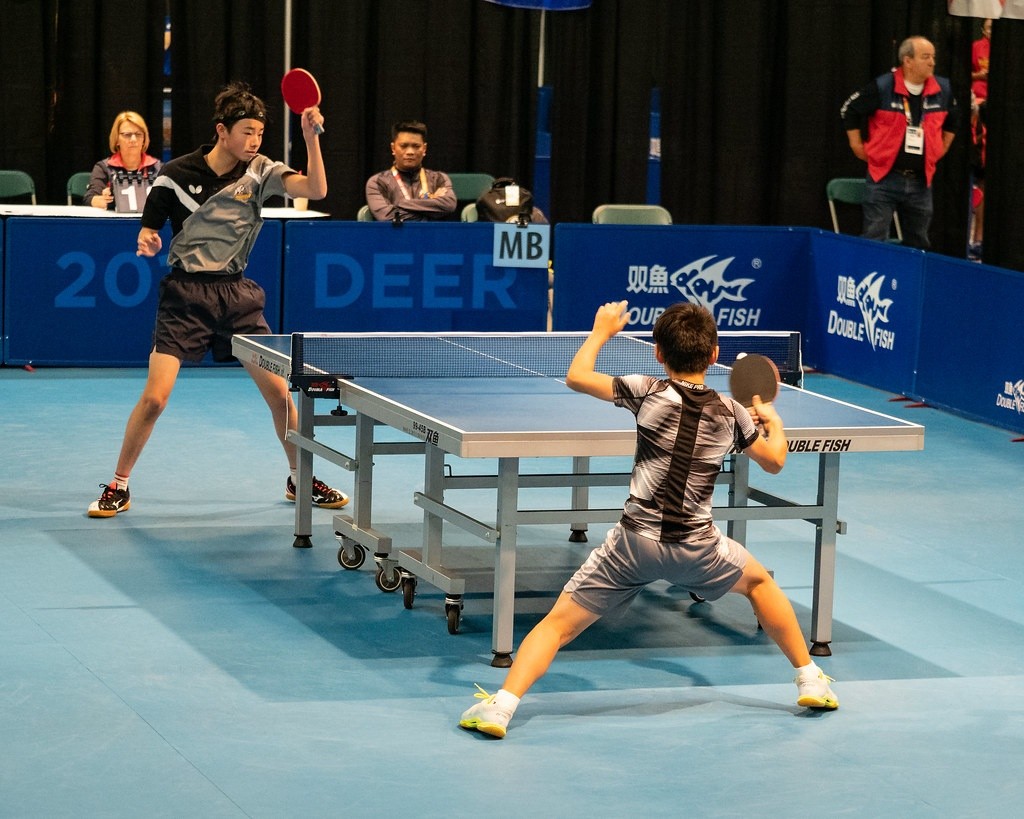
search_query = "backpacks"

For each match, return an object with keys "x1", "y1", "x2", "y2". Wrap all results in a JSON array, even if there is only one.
[{"x1": 476, "y1": 177, "x2": 534, "y2": 222}]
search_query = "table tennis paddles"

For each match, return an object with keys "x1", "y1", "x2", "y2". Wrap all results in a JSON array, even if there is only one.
[
  {"x1": 729, "y1": 353, "x2": 781, "y2": 437},
  {"x1": 281, "y1": 68, "x2": 325, "y2": 135}
]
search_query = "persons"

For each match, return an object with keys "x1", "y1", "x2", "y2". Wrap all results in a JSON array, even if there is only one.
[
  {"x1": 85, "y1": 112, "x2": 164, "y2": 214},
  {"x1": 842, "y1": 36, "x2": 955, "y2": 249},
  {"x1": 365, "y1": 121, "x2": 456, "y2": 223},
  {"x1": 87, "y1": 84, "x2": 347, "y2": 516},
  {"x1": 459, "y1": 299, "x2": 839, "y2": 739},
  {"x1": 968, "y1": 18, "x2": 993, "y2": 257}
]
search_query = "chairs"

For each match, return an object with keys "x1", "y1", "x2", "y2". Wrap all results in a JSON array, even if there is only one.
[
  {"x1": 593, "y1": 204, "x2": 672, "y2": 226},
  {"x1": 67, "y1": 172, "x2": 91, "y2": 205},
  {"x1": 0, "y1": 170, "x2": 36, "y2": 205},
  {"x1": 445, "y1": 174, "x2": 495, "y2": 201},
  {"x1": 827, "y1": 178, "x2": 904, "y2": 245}
]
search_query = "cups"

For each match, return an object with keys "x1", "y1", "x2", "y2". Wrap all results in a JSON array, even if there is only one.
[{"x1": 292, "y1": 196, "x2": 308, "y2": 211}]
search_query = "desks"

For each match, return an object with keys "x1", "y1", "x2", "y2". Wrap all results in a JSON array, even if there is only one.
[
  {"x1": 0, "y1": 204, "x2": 333, "y2": 221},
  {"x1": 230, "y1": 332, "x2": 926, "y2": 668}
]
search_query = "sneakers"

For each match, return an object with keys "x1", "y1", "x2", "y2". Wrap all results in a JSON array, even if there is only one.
[
  {"x1": 795, "y1": 668, "x2": 839, "y2": 708},
  {"x1": 460, "y1": 683, "x2": 513, "y2": 737},
  {"x1": 286, "y1": 475, "x2": 349, "y2": 508},
  {"x1": 88, "y1": 481, "x2": 130, "y2": 517}
]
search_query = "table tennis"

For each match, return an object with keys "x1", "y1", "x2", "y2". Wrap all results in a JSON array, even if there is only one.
[{"x1": 736, "y1": 352, "x2": 748, "y2": 359}]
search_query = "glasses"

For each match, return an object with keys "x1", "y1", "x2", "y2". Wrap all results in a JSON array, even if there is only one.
[{"x1": 119, "y1": 132, "x2": 145, "y2": 137}]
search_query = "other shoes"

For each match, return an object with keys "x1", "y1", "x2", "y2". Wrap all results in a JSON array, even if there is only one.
[{"x1": 970, "y1": 241, "x2": 983, "y2": 257}]
